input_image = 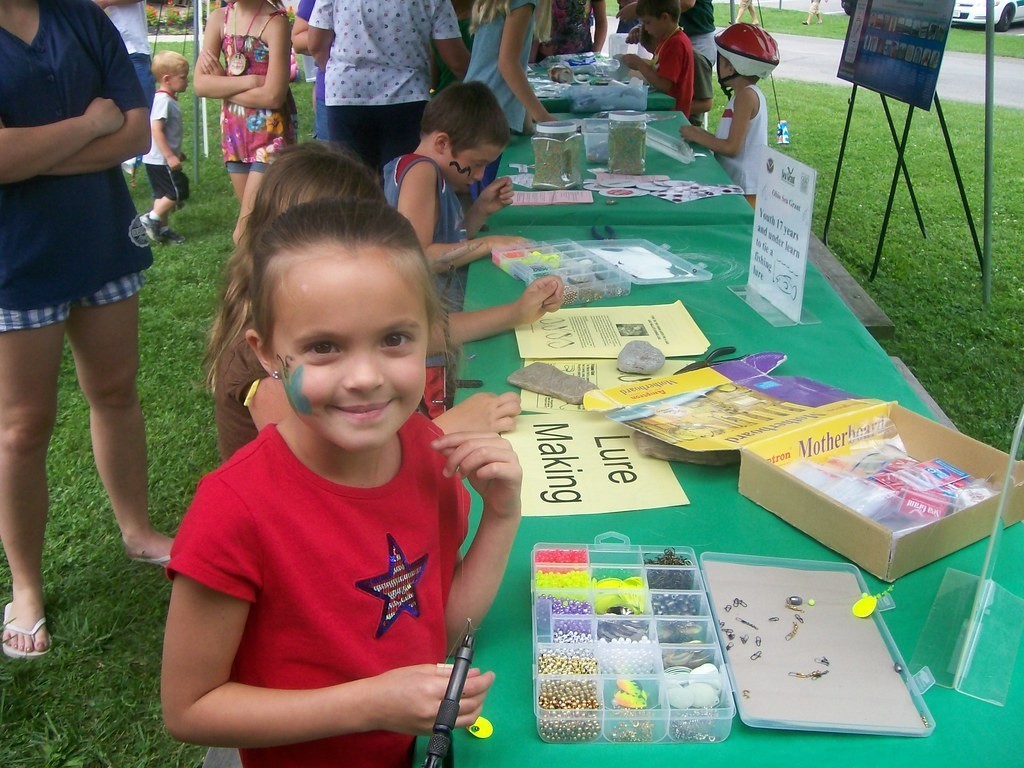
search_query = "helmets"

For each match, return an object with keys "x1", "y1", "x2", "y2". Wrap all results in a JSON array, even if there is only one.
[{"x1": 714, "y1": 23, "x2": 780, "y2": 79}]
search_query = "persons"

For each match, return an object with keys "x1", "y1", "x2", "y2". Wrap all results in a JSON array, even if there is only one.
[
  {"x1": 0, "y1": 0, "x2": 175, "y2": 660},
  {"x1": 161, "y1": 199, "x2": 523, "y2": 768},
  {"x1": 94, "y1": 0, "x2": 780, "y2": 455},
  {"x1": 802, "y1": 0, "x2": 828, "y2": 26}
]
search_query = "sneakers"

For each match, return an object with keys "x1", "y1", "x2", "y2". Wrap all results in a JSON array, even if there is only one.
[{"x1": 139, "y1": 212, "x2": 185, "y2": 244}]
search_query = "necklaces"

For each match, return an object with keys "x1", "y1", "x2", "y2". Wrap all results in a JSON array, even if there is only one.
[{"x1": 229, "y1": 1, "x2": 263, "y2": 75}]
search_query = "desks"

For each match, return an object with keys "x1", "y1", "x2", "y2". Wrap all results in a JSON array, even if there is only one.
[{"x1": 417, "y1": 57, "x2": 1024, "y2": 768}]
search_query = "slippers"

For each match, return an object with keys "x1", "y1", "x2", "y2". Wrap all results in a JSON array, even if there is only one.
[
  {"x1": 129, "y1": 554, "x2": 171, "y2": 568},
  {"x1": 3, "y1": 602, "x2": 52, "y2": 660}
]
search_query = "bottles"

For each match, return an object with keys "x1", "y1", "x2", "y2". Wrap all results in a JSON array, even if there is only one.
[
  {"x1": 531, "y1": 121, "x2": 584, "y2": 191},
  {"x1": 607, "y1": 110, "x2": 648, "y2": 175}
]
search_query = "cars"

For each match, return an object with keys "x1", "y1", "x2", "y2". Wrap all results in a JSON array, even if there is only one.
[
  {"x1": 841, "y1": 0, "x2": 853, "y2": 15},
  {"x1": 952, "y1": 0, "x2": 1024, "y2": 32}
]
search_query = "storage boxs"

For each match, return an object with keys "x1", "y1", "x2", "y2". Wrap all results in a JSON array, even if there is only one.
[
  {"x1": 581, "y1": 118, "x2": 696, "y2": 165},
  {"x1": 582, "y1": 353, "x2": 1024, "y2": 584},
  {"x1": 531, "y1": 532, "x2": 937, "y2": 742},
  {"x1": 566, "y1": 86, "x2": 649, "y2": 113},
  {"x1": 491, "y1": 238, "x2": 713, "y2": 306}
]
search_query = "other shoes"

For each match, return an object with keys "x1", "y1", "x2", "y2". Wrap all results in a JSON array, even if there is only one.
[{"x1": 802, "y1": 21, "x2": 809, "y2": 25}]
